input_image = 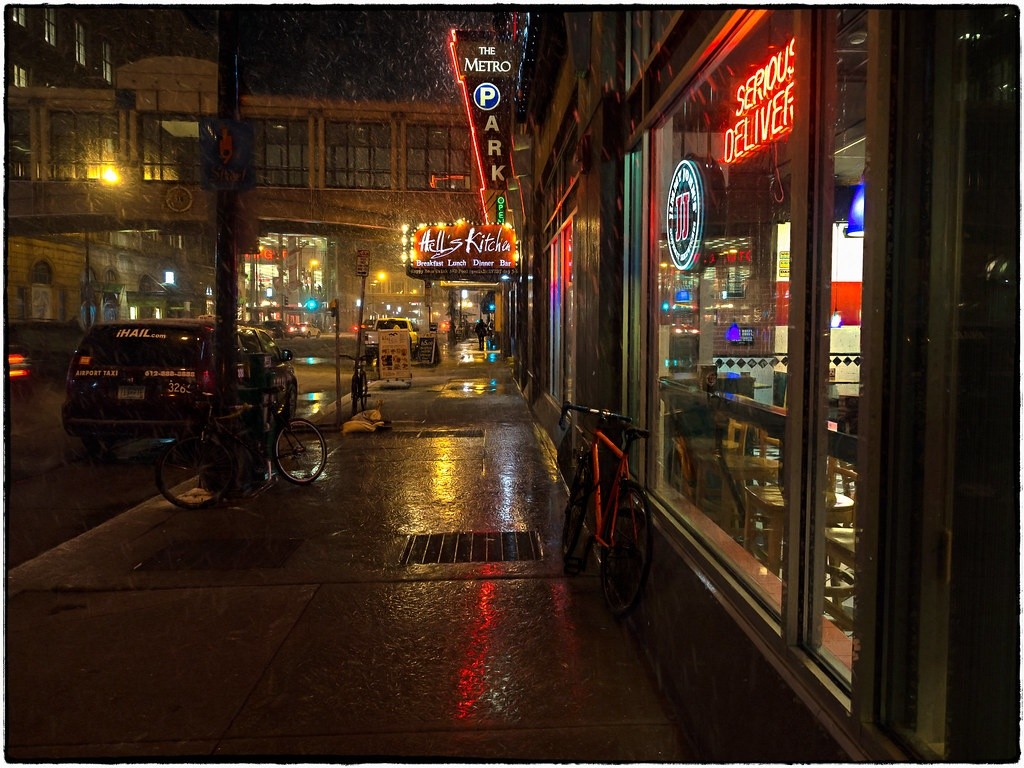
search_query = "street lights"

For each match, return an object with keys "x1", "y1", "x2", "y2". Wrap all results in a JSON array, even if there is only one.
[{"x1": 84, "y1": 167, "x2": 120, "y2": 330}]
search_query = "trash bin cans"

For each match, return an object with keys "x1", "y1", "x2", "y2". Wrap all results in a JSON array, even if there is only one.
[{"x1": 238, "y1": 351, "x2": 277, "y2": 485}]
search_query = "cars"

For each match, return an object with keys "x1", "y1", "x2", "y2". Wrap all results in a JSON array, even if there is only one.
[
  {"x1": 261, "y1": 319, "x2": 289, "y2": 339},
  {"x1": 287, "y1": 323, "x2": 322, "y2": 339},
  {"x1": 59, "y1": 318, "x2": 298, "y2": 474}
]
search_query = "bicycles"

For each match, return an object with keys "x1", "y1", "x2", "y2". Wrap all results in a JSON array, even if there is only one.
[
  {"x1": 153, "y1": 387, "x2": 327, "y2": 510},
  {"x1": 557, "y1": 399, "x2": 654, "y2": 615},
  {"x1": 351, "y1": 354, "x2": 372, "y2": 416}
]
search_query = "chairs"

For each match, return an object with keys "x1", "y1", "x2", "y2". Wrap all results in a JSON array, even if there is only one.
[{"x1": 668, "y1": 410, "x2": 860, "y2": 645}]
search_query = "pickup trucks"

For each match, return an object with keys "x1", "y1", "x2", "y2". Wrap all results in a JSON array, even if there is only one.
[{"x1": 364, "y1": 317, "x2": 419, "y2": 364}]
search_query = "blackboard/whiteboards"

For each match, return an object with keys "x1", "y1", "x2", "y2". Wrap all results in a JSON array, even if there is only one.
[{"x1": 417, "y1": 337, "x2": 436, "y2": 363}]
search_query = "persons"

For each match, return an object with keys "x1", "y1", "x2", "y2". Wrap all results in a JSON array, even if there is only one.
[
  {"x1": 475, "y1": 319, "x2": 488, "y2": 351},
  {"x1": 488, "y1": 319, "x2": 493, "y2": 339}
]
